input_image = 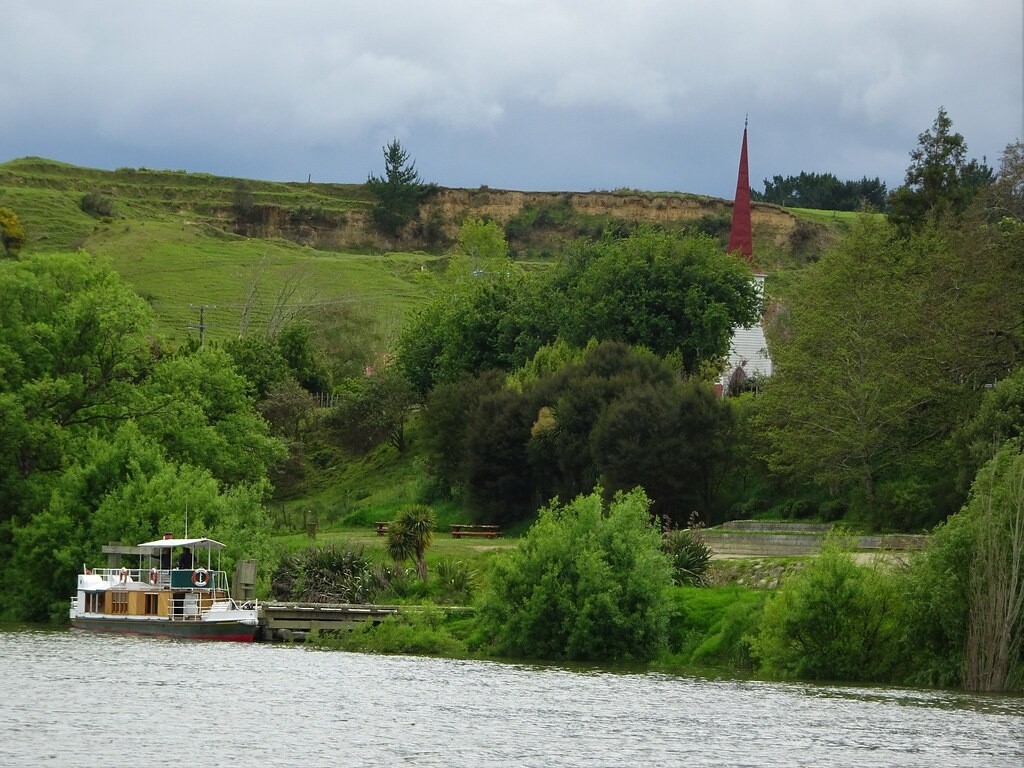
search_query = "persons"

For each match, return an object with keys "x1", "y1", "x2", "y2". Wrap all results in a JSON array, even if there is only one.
[
  {"x1": 176, "y1": 548, "x2": 195, "y2": 569},
  {"x1": 151, "y1": 548, "x2": 171, "y2": 570}
]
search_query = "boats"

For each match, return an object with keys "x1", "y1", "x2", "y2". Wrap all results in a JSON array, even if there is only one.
[{"x1": 69, "y1": 532, "x2": 259, "y2": 643}]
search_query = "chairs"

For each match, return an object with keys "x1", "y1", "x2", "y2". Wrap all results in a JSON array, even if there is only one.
[{"x1": 183, "y1": 593, "x2": 200, "y2": 616}]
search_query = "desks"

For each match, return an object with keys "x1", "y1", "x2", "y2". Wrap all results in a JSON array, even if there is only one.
[
  {"x1": 451, "y1": 523, "x2": 503, "y2": 538},
  {"x1": 374, "y1": 521, "x2": 393, "y2": 537}
]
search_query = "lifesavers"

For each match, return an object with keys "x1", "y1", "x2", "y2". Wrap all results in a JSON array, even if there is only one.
[
  {"x1": 119, "y1": 567, "x2": 127, "y2": 583},
  {"x1": 192, "y1": 568, "x2": 210, "y2": 587},
  {"x1": 150, "y1": 568, "x2": 158, "y2": 584}
]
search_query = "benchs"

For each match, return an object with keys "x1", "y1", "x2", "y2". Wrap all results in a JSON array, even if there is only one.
[{"x1": 452, "y1": 531, "x2": 498, "y2": 536}]
